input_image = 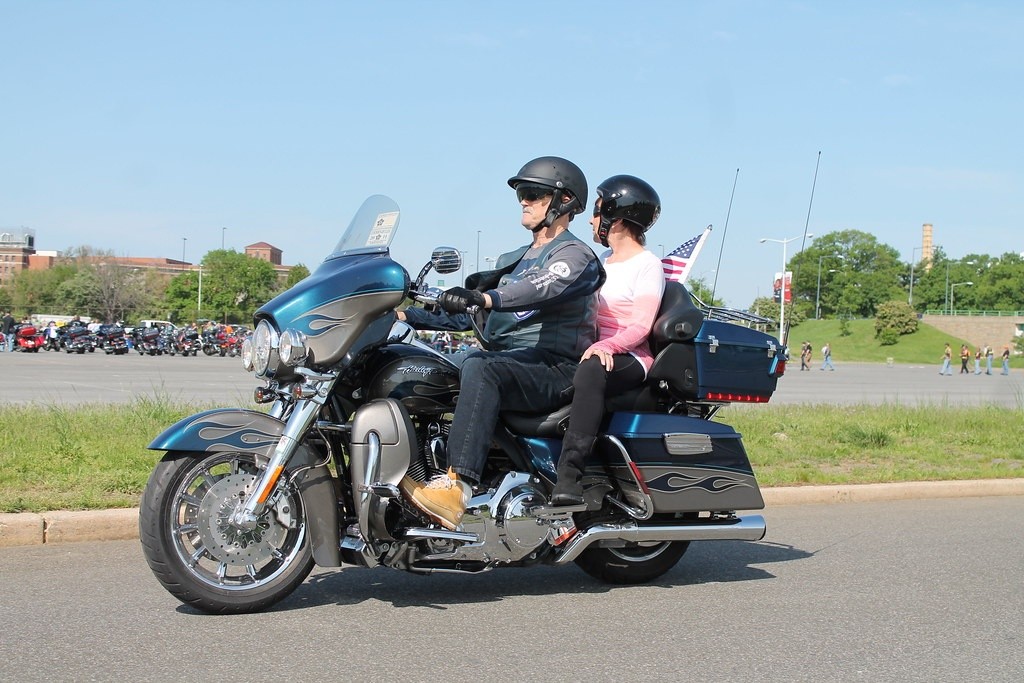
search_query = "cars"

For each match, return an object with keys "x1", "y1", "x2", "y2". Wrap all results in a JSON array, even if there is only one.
[{"x1": 431, "y1": 332, "x2": 472, "y2": 354}]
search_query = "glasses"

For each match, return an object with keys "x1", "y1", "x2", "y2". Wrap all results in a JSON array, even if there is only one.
[
  {"x1": 593, "y1": 204, "x2": 601, "y2": 217},
  {"x1": 518, "y1": 188, "x2": 555, "y2": 202}
]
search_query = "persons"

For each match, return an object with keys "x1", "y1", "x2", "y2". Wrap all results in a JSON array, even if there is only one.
[
  {"x1": 550, "y1": 175, "x2": 666, "y2": 506},
  {"x1": 939, "y1": 343, "x2": 1009, "y2": 376},
  {"x1": 801, "y1": 341, "x2": 835, "y2": 371},
  {"x1": 0, "y1": 310, "x2": 253, "y2": 358},
  {"x1": 394, "y1": 157, "x2": 606, "y2": 532}
]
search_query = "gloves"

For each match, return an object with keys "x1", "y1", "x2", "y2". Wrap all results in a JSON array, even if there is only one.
[{"x1": 438, "y1": 286, "x2": 487, "y2": 314}]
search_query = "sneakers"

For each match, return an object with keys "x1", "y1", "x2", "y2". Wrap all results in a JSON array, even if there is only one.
[{"x1": 399, "y1": 466, "x2": 473, "y2": 532}]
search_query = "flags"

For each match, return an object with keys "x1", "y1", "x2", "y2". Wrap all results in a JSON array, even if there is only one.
[{"x1": 661, "y1": 234, "x2": 707, "y2": 283}]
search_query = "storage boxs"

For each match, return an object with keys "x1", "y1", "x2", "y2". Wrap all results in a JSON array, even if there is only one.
[
  {"x1": 694, "y1": 320, "x2": 785, "y2": 402},
  {"x1": 609, "y1": 411, "x2": 765, "y2": 512}
]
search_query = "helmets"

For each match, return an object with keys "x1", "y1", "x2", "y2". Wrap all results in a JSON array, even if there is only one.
[
  {"x1": 507, "y1": 156, "x2": 588, "y2": 217},
  {"x1": 596, "y1": 174, "x2": 662, "y2": 232}
]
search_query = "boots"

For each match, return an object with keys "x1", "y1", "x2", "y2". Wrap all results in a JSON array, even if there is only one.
[{"x1": 550, "y1": 428, "x2": 598, "y2": 507}]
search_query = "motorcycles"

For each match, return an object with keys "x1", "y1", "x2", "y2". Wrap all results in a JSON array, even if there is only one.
[
  {"x1": 0, "y1": 313, "x2": 255, "y2": 359},
  {"x1": 136, "y1": 193, "x2": 791, "y2": 614}
]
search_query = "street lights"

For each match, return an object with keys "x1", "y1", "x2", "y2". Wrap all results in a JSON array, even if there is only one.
[
  {"x1": 222, "y1": 226, "x2": 227, "y2": 250},
  {"x1": 476, "y1": 230, "x2": 482, "y2": 273},
  {"x1": 950, "y1": 282, "x2": 973, "y2": 316},
  {"x1": 829, "y1": 269, "x2": 855, "y2": 319},
  {"x1": 485, "y1": 257, "x2": 497, "y2": 269},
  {"x1": 758, "y1": 232, "x2": 815, "y2": 346},
  {"x1": 182, "y1": 238, "x2": 188, "y2": 273},
  {"x1": 814, "y1": 254, "x2": 845, "y2": 320},
  {"x1": 197, "y1": 264, "x2": 205, "y2": 319},
  {"x1": 459, "y1": 250, "x2": 468, "y2": 288},
  {"x1": 909, "y1": 245, "x2": 937, "y2": 306},
  {"x1": 944, "y1": 261, "x2": 974, "y2": 314}
]
